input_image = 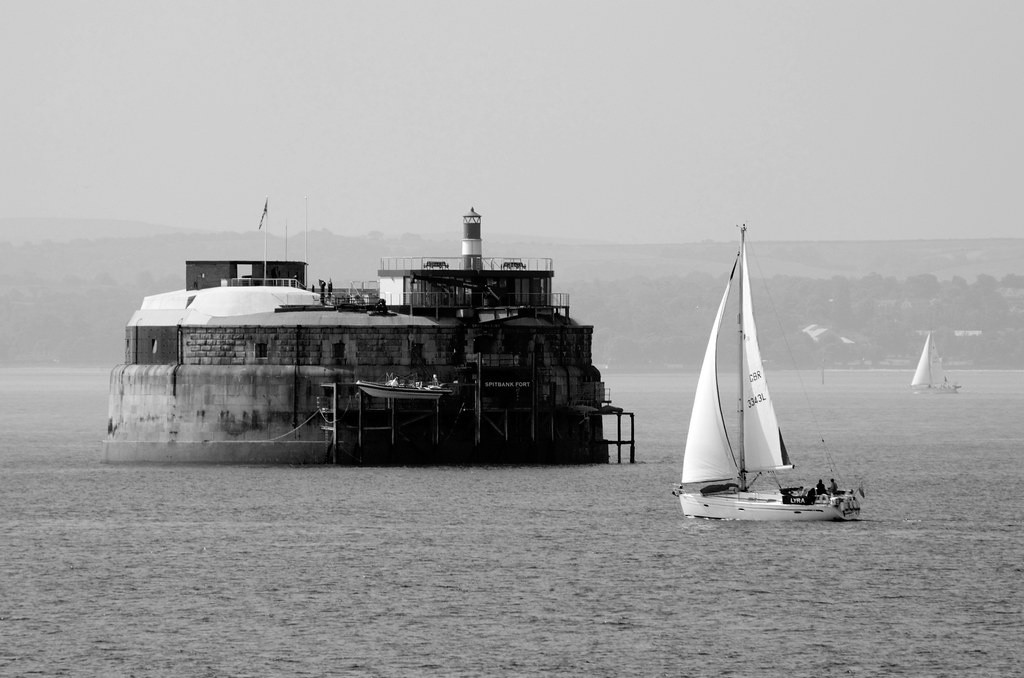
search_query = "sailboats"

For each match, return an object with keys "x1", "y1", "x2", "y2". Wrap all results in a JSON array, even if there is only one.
[
  {"x1": 910, "y1": 330, "x2": 962, "y2": 394},
  {"x1": 672, "y1": 224, "x2": 862, "y2": 521}
]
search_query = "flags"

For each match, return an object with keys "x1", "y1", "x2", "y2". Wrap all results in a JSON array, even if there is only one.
[{"x1": 259, "y1": 200, "x2": 267, "y2": 229}]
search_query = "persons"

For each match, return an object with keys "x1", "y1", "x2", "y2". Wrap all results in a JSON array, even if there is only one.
[
  {"x1": 800, "y1": 486, "x2": 815, "y2": 504},
  {"x1": 817, "y1": 479, "x2": 825, "y2": 495},
  {"x1": 829, "y1": 479, "x2": 838, "y2": 494}
]
